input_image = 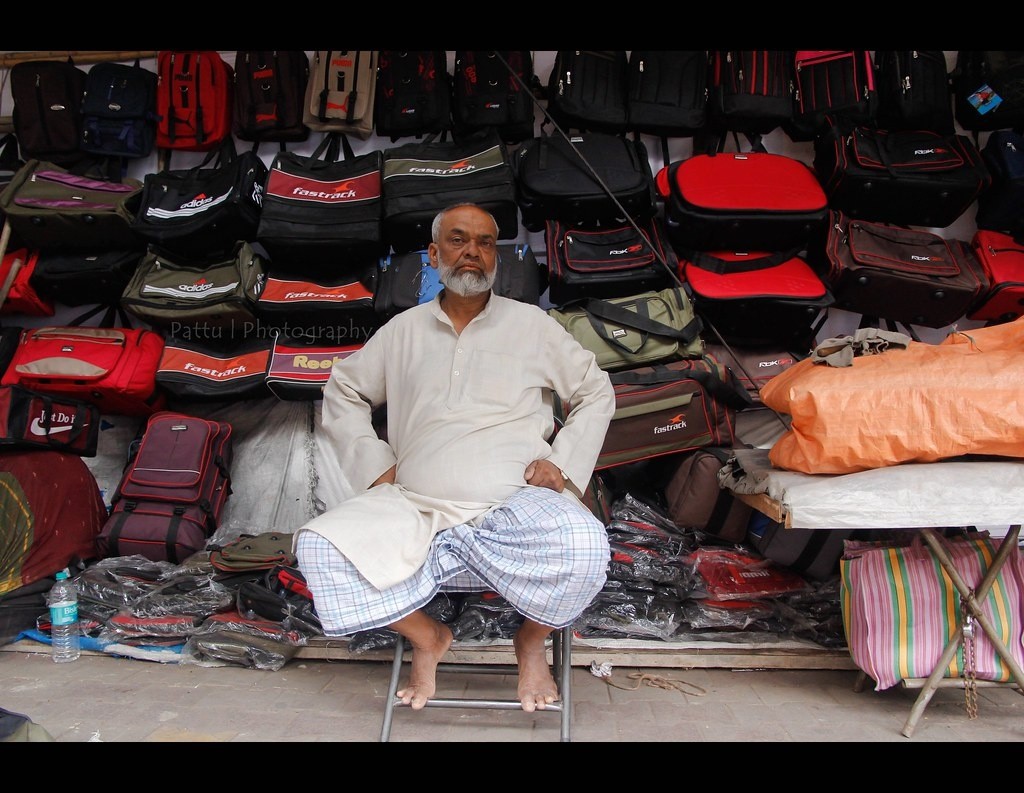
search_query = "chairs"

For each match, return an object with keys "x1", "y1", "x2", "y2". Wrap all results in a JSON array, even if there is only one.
[{"x1": 377, "y1": 415, "x2": 578, "y2": 743}]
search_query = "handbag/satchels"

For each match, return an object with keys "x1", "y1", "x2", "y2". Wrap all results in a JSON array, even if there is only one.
[
  {"x1": 206, "y1": 526, "x2": 301, "y2": 572},
  {"x1": 839, "y1": 536, "x2": 1024, "y2": 691},
  {"x1": 1, "y1": 131, "x2": 1023, "y2": 469},
  {"x1": 751, "y1": 494, "x2": 855, "y2": 578},
  {"x1": 666, "y1": 450, "x2": 751, "y2": 543}
]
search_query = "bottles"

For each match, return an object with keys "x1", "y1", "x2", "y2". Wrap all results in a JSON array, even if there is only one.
[{"x1": 47, "y1": 573, "x2": 81, "y2": 664}]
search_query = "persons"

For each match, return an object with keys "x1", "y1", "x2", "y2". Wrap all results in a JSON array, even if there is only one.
[{"x1": 291, "y1": 202, "x2": 616, "y2": 715}]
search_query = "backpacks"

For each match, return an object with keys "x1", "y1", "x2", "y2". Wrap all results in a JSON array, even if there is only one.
[
  {"x1": 11, "y1": 49, "x2": 1024, "y2": 164},
  {"x1": 99, "y1": 409, "x2": 234, "y2": 564}
]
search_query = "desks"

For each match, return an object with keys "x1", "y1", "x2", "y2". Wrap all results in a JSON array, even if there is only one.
[{"x1": 722, "y1": 450, "x2": 1024, "y2": 738}]
